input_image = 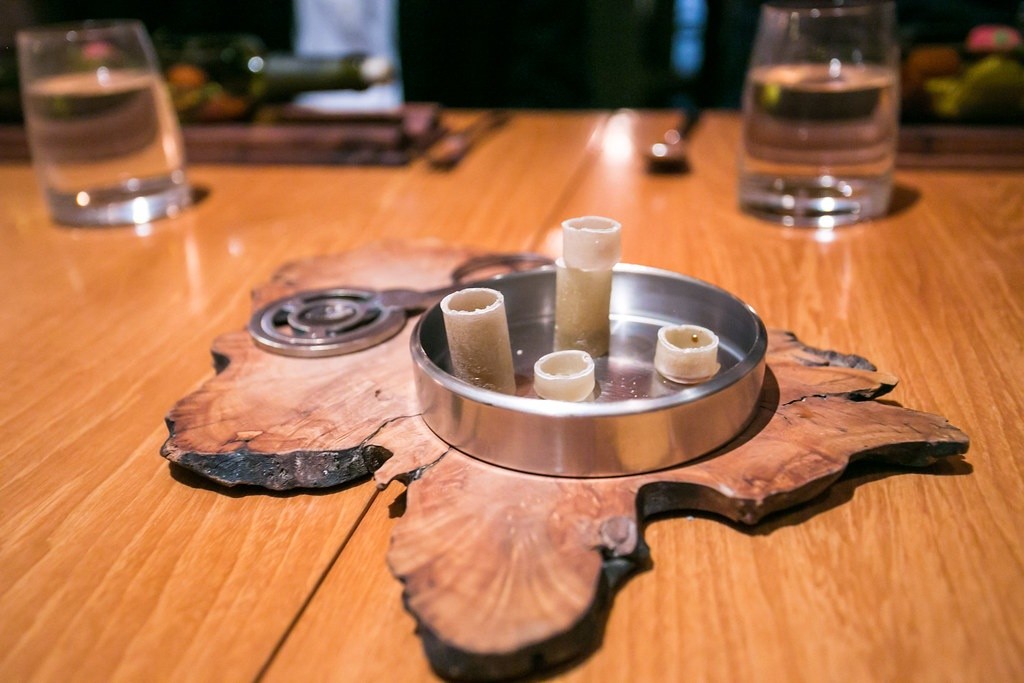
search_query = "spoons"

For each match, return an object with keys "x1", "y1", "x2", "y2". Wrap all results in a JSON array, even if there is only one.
[{"x1": 643, "y1": 104, "x2": 704, "y2": 162}]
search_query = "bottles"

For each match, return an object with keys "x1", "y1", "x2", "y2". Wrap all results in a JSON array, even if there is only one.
[{"x1": 3, "y1": 33, "x2": 391, "y2": 129}]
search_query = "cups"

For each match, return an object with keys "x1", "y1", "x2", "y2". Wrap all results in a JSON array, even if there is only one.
[
  {"x1": 14, "y1": 25, "x2": 186, "y2": 228},
  {"x1": 736, "y1": 0, "x2": 899, "y2": 230}
]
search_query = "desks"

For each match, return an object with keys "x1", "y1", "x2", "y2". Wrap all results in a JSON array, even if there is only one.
[{"x1": 0, "y1": 104, "x2": 1023, "y2": 683}]
